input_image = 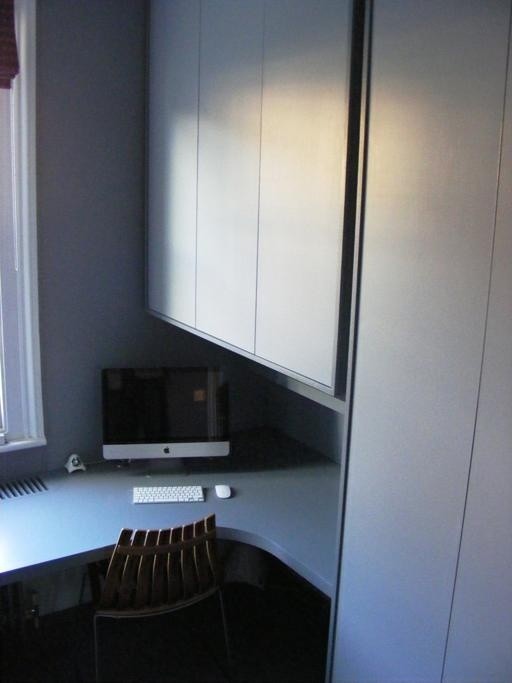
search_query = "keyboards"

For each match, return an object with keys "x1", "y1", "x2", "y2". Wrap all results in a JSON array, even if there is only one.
[{"x1": 133, "y1": 486, "x2": 204, "y2": 504}]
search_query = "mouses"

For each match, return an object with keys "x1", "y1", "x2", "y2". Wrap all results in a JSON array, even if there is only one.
[{"x1": 215, "y1": 485, "x2": 231, "y2": 498}]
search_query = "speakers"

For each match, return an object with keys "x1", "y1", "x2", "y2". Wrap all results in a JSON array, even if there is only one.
[{"x1": 64, "y1": 453, "x2": 86, "y2": 474}]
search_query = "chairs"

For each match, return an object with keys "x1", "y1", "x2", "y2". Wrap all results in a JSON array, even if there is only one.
[{"x1": 92, "y1": 513, "x2": 234, "y2": 682}]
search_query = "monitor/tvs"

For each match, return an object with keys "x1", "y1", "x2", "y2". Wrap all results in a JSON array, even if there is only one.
[{"x1": 101, "y1": 366, "x2": 230, "y2": 477}]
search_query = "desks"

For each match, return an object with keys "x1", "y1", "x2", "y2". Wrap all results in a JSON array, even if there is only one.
[{"x1": 0, "y1": 430, "x2": 345, "y2": 682}]
